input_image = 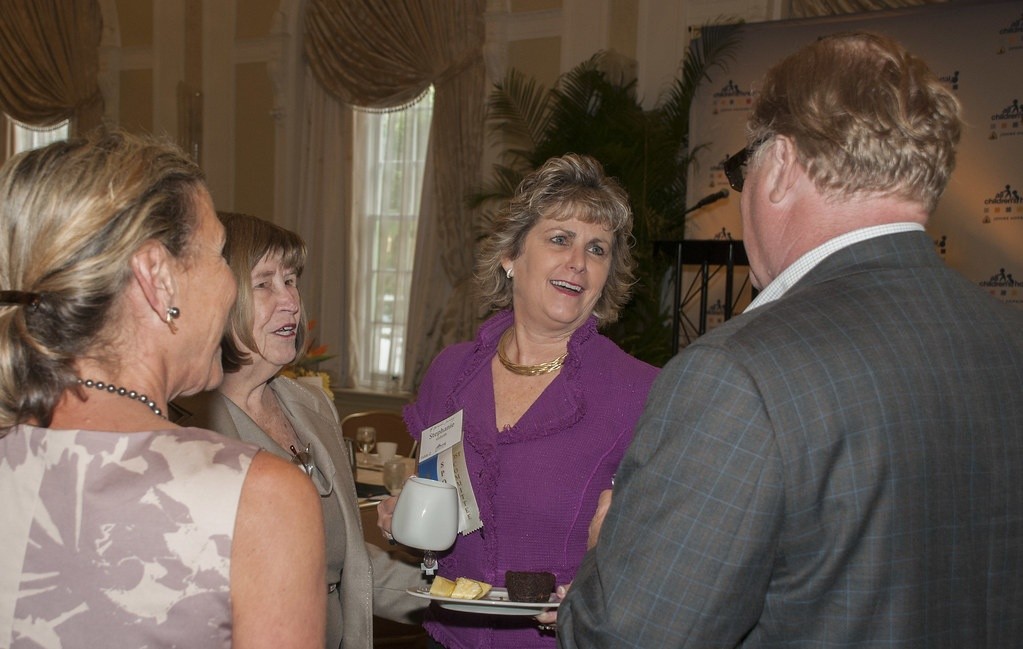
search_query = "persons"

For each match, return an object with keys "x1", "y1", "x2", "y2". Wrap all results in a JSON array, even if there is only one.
[
  {"x1": 171, "y1": 211, "x2": 431, "y2": 649},
  {"x1": 557, "y1": 35, "x2": 1023, "y2": 649},
  {"x1": 0, "y1": 130, "x2": 327, "y2": 648},
  {"x1": 378, "y1": 154, "x2": 661, "y2": 649}
]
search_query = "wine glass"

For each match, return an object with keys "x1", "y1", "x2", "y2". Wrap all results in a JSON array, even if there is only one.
[{"x1": 356, "y1": 427, "x2": 376, "y2": 468}]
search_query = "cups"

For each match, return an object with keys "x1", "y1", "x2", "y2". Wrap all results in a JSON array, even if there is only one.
[
  {"x1": 382, "y1": 456, "x2": 415, "y2": 497},
  {"x1": 391, "y1": 475, "x2": 459, "y2": 551},
  {"x1": 375, "y1": 441, "x2": 398, "y2": 466}
]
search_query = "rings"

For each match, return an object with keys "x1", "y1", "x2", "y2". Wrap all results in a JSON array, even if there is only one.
[{"x1": 537, "y1": 625, "x2": 553, "y2": 631}]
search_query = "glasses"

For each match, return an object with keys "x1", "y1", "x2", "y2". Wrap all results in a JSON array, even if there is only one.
[
  {"x1": 290, "y1": 444, "x2": 332, "y2": 496},
  {"x1": 724, "y1": 132, "x2": 775, "y2": 190}
]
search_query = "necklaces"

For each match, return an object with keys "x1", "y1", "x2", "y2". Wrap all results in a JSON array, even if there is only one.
[
  {"x1": 76, "y1": 379, "x2": 167, "y2": 419},
  {"x1": 498, "y1": 327, "x2": 568, "y2": 375}
]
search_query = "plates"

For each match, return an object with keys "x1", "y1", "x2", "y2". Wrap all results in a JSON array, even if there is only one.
[{"x1": 404, "y1": 584, "x2": 562, "y2": 618}]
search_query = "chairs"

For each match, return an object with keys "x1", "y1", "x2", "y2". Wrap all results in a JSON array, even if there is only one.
[{"x1": 340, "y1": 411, "x2": 418, "y2": 458}]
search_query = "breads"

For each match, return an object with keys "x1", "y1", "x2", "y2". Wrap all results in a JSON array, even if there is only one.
[
  {"x1": 430, "y1": 576, "x2": 492, "y2": 600},
  {"x1": 505, "y1": 570, "x2": 556, "y2": 603}
]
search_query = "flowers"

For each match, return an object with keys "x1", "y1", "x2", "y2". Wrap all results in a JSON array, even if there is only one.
[{"x1": 276, "y1": 323, "x2": 335, "y2": 402}]
